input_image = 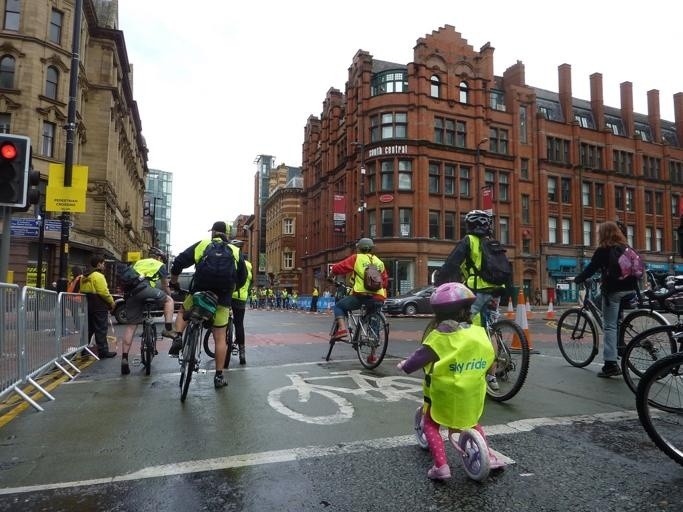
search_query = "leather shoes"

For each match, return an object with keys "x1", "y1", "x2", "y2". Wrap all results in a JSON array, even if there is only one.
[{"x1": 596, "y1": 360, "x2": 621, "y2": 378}]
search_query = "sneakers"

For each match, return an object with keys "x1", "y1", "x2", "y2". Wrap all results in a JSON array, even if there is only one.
[
  {"x1": 119, "y1": 358, "x2": 129, "y2": 375},
  {"x1": 166, "y1": 331, "x2": 183, "y2": 354},
  {"x1": 329, "y1": 328, "x2": 350, "y2": 340},
  {"x1": 160, "y1": 328, "x2": 179, "y2": 339},
  {"x1": 484, "y1": 449, "x2": 506, "y2": 471},
  {"x1": 486, "y1": 378, "x2": 499, "y2": 391},
  {"x1": 365, "y1": 353, "x2": 379, "y2": 364},
  {"x1": 97, "y1": 348, "x2": 117, "y2": 360},
  {"x1": 212, "y1": 375, "x2": 228, "y2": 388},
  {"x1": 425, "y1": 463, "x2": 453, "y2": 482},
  {"x1": 237, "y1": 343, "x2": 247, "y2": 364}
]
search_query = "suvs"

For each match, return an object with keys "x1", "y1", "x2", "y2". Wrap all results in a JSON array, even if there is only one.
[{"x1": 107, "y1": 270, "x2": 193, "y2": 325}]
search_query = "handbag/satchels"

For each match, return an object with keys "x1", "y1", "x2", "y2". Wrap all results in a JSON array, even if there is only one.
[{"x1": 114, "y1": 264, "x2": 144, "y2": 294}]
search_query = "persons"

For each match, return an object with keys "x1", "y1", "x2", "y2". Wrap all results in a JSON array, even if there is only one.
[
  {"x1": 249, "y1": 284, "x2": 299, "y2": 311},
  {"x1": 323, "y1": 287, "x2": 332, "y2": 298},
  {"x1": 534, "y1": 286, "x2": 543, "y2": 303},
  {"x1": 81, "y1": 258, "x2": 118, "y2": 359},
  {"x1": 430, "y1": 210, "x2": 507, "y2": 396},
  {"x1": 229, "y1": 237, "x2": 252, "y2": 363},
  {"x1": 311, "y1": 286, "x2": 318, "y2": 313},
  {"x1": 398, "y1": 285, "x2": 516, "y2": 480},
  {"x1": 326, "y1": 237, "x2": 389, "y2": 367},
  {"x1": 556, "y1": 284, "x2": 562, "y2": 306},
  {"x1": 563, "y1": 221, "x2": 645, "y2": 377},
  {"x1": 66, "y1": 264, "x2": 81, "y2": 336},
  {"x1": 119, "y1": 244, "x2": 177, "y2": 374},
  {"x1": 167, "y1": 220, "x2": 247, "y2": 388}
]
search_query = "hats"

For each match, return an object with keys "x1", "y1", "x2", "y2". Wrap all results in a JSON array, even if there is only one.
[
  {"x1": 207, "y1": 221, "x2": 233, "y2": 235},
  {"x1": 354, "y1": 237, "x2": 373, "y2": 251}
]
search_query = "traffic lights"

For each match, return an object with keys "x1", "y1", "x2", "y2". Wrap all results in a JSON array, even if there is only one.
[
  {"x1": 13, "y1": 145, "x2": 41, "y2": 213},
  {"x1": 0, "y1": 133, "x2": 31, "y2": 210}
]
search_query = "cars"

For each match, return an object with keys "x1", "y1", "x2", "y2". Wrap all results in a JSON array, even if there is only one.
[{"x1": 379, "y1": 283, "x2": 435, "y2": 318}]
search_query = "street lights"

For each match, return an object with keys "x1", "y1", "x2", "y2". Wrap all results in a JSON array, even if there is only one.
[
  {"x1": 476, "y1": 137, "x2": 487, "y2": 213},
  {"x1": 163, "y1": 243, "x2": 172, "y2": 272},
  {"x1": 151, "y1": 196, "x2": 162, "y2": 247},
  {"x1": 350, "y1": 141, "x2": 365, "y2": 240}
]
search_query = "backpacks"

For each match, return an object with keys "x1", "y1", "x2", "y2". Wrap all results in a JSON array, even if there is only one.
[
  {"x1": 467, "y1": 234, "x2": 512, "y2": 286},
  {"x1": 64, "y1": 275, "x2": 83, "y2": 305},
  {"x1": 195, "y1": 239, "x2": 235, "y2": 293},
  {"x1": 353, "y1": 253, "x2": 383, "y2": 292},
  {"x1": 611, "y1": 243, "x2": 645, "y2": 283}
]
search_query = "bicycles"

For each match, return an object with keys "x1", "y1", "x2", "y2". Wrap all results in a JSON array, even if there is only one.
[
  {"x1": 320, "y1": 273, "x2": 390, "y2": 370},
  {"x1": 553, "y1": 260, "x2": 683, "y2": 467},
  {"x1": 126, "y1": 283, "x2": 239, "y2": 404},
  {"x1": 417, "y1": 265, "x2": 529, "y2": 403},
  {"x1": 397, "y1": 356, "x2": 490, "y2": 484}
]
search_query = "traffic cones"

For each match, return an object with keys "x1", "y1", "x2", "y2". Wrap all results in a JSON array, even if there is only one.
[{"x1": 497, "y1": 285, "x2": 562, "y2": 354}]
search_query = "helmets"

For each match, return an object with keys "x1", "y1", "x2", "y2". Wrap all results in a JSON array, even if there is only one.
[
  {"x1": 147, "y1": 246, "x2": 168, "y2": 264},
  {"x1": 228, "y1": 238, "x2": 243, "y2": 248},
  {"x1": 428, "y1": 281, "x2": 477, "y2": 308},
  {"x1": 464, "y1": 209, "x2": 490, "y2": 225}
]
